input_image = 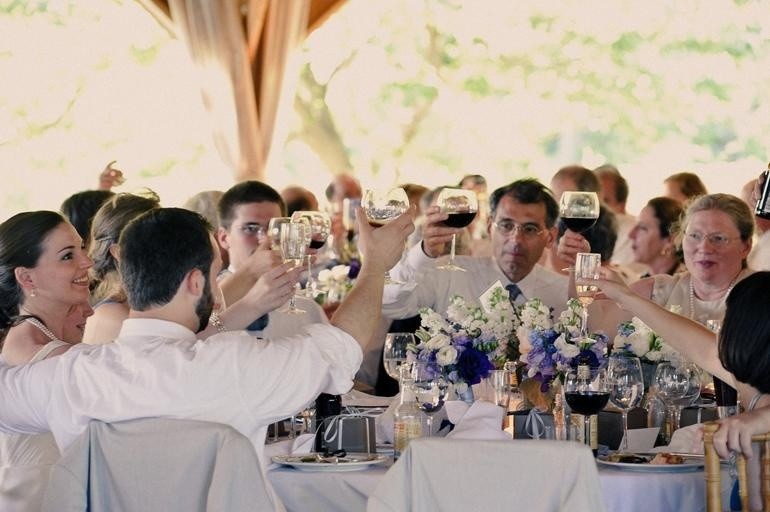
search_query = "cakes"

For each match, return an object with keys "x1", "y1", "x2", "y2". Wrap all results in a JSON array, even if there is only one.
[{"x1": 568, "y1": 364, "x2": 600, "y2": 458}]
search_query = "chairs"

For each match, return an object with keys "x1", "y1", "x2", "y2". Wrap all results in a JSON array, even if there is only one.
[
  {"x1": 410, "y1": 439, "x2": 594, "y2": 512},
  {"x1": 83, "y1": 419, "x2": 281, "y2": 512},
  {"x1": 704, "y1": 421, "x2": 770, "y2": 511}
]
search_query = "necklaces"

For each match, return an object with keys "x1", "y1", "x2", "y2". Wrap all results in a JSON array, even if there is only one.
[
  {"x1": 689, "y1": 274, "x2": 738, "y2": 324},
  {"x1": 19, "y1": 302, "x2": 60, "y2": 342}
]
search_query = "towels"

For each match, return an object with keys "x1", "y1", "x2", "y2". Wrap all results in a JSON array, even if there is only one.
[{"x1": 443, "y1": 400, "x2": 513, "y2": 441}]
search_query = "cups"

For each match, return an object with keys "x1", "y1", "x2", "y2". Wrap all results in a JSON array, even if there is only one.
[
  {"x1": 564, "y1": 368, "x2": 609, "y2": 446},
  {"x1": 609, "y1": 356, "x2": 644, "y2": 431},
  {"x1": 361, "y1": 188, "x2": 410, "y2": 285},
  {"x1": 412, "y1": 361, "x2": 448, "y2": 437},
  {"x1": 571, "y1": 253, "x2": 602, "y2": 342},
  {"x1": 384, "y1": 332, "x2": 416, "y2": 383},
  {"x1": 268, "y1": 217, "x2": 312, "y2": 251},
  {"x1": 655, "y1": 362, "x2": 689, "y2": 442},
  {"x1": 664, "y1": 362, "x2": 703, "y2": 430},
  {"x1": 282, "y1": 224, "x2": 306, "y2": 315},
  {"x1": 342, "y1": 199, "x2": 364, "y2": 242},
  {"x1": 291, "y1": 211, "x2": 332, "y2": 296},
  {"x1": 559, "y1": 191, "x2": 599, "y2": 271},
  {"x1": 486, "y1": 369, "x2": 510, "y2": 409},
  {"x1": 434, "y1": 188, "x2": 477, "y2": 273}
]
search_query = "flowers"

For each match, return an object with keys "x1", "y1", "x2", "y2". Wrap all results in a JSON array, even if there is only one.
[
  {"x1": 407, "y1": 299, "x2": 502, "y2": 389},
  {"x1": 301, "y1": 264, "x2": 357, "y2": 309},
  {"x1": 520, "y1": 299, "x2": 609, "y2": 421},
  {"x1": 610, "y1": 305, "x2": 703, "y2": 373}
]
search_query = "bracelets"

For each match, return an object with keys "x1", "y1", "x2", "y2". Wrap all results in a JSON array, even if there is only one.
[{"x1": 208, "y1": 312, "x2": 227, "y2": 333}]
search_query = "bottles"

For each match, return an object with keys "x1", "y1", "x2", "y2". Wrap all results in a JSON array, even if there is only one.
[
  {"x1": 392, "y1": 376, "x2": 424, "y2": 462},
  {"x1": 314, "y1": 392, "x2": 343, "y2": 453},
  {"x1": 552, "y1": 394, "x2": 567, "y2": 441},
  {"x1": 646, "y1": 353, "x2": 679, "y2": 446},
  {"x1": 503, "y1": 361, "x2": 525, "y2": 411}
]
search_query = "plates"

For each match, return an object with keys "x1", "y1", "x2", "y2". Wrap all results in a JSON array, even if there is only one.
[
  {"x1": 596, "y1": 454, "x2": 704, "y2": 472},
  {"x1": 272, "y1": 453, "x2": 389, "y2": 472}
]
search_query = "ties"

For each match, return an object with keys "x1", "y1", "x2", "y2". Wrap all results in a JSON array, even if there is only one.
[{"x1": 504, "y1": 285, "x2": 522, "y2": 303}]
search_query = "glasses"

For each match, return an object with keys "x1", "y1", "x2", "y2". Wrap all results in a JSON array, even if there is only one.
[
  {"x1": 684, "y1": 229, "x2": 741, "y2": 246},
  {"x1": 239, "y1": 224, "x2": 262, "y2": 236},
  {"x1": 490, "y1": 217, "x2": 544, "y2": 240}
]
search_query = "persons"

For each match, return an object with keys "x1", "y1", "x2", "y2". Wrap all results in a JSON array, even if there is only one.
[
  {"x1": 555, "y1": 193, "x2": 755, "y2": 343},
  {"x1": 756, "y1": 164, "x2": 770, "y2": 219},
  {"x1": 82, "y1": 191, "x2": 282, "y2": 346},
  {"x1": 549, "y1": 165, "x2": 638, "y2": 268},
  {"x1": 0, "y1": 204, "x2": 416, "y2": 512},
  {"x1": 181, "y1": 190, "x2": 229, "y2": 278},
  {"x1": 578, "y1": 265, "x2": 770, "y2": 512},
  {"x1": 62, "y1": 160, "x2": 124, "y2": 253},
  {"x1": 0, "y1": 211, "x2": 301, "y2": 466},
  {"x1": 390, "y1": 180, "x2": 570, "y2": 322},
  {"x1": 392, "y1": 166, "x2": 489, "y2": 259},
  {"x1": 217, "y1": 180, "x2": 329, "y2": 338},
  {"x1": 592, "y1": 163, "x2": 636, "y2": 216},
  {"x1": 740, "y1": 179, "x2": 769, "y2": 270},
  {"x1": 661, "y1": 172, "x2": 708, "y2": 200},
  {"x1": 325, "y1": 173, "x2": 385, "y2": 241},
  {"x1": 278, "y1": 186, "x2": 318, "y2": 218},
  {"x1": 618, "y1": 197, "x2": 687, "y2": 276}
]
